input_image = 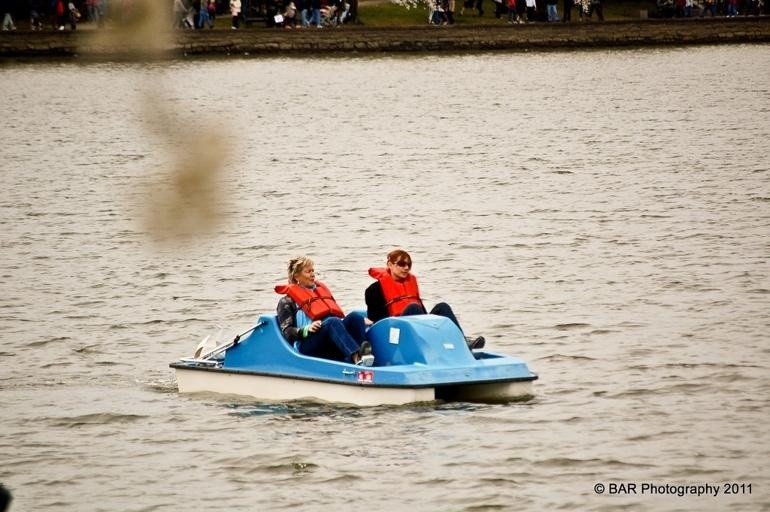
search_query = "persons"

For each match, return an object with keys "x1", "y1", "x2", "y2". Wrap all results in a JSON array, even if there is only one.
[
  {"x1": 273, "y1": 256, "x2": 376, "y2": 365},
  {"x1": 0, "y1": 1, "x2": 769, "y2": 33},
  {"x1": 363, "y1": 249, "x2": 487, "y2": 359}
]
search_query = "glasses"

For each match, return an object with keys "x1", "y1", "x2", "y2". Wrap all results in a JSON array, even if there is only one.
[{"x1": 392, "y1": 260, "x2": 411, "y2": 268}]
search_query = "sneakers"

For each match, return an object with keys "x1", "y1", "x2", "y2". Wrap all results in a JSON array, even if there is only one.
[
  {"x1": 465, "y1": 337, "x2": 484, "y2": 350},
  {"x1": 356, "y1": 341, "x2": 373, "y2": 361}
]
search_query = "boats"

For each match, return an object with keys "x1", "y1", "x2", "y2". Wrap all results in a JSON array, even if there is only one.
[{"x1": 169, "y1": 310, "x2": 538, "y2": 406}]
